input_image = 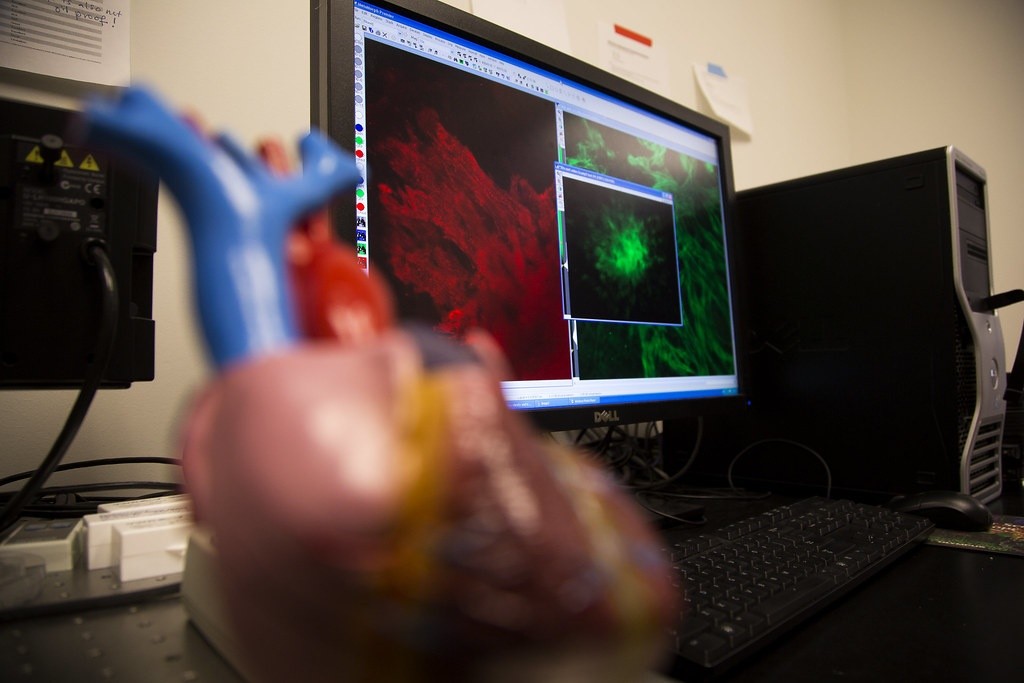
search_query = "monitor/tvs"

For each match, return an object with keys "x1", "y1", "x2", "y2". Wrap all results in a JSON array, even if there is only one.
[
  {"x1": 0, "y1": 65, "x2": 159, "y2": 389},
  {"x1": 311, "y1": 0, "x2": 755, "y2": 432}
]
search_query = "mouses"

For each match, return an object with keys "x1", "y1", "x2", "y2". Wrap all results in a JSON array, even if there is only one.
[{"x1": 886, "y1": 490, "x2": 994, "y2": 533}]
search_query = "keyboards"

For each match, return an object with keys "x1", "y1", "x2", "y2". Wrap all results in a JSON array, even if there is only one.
[{"x1": 660, "y1": 495, "x2": 936, "y2": 677}]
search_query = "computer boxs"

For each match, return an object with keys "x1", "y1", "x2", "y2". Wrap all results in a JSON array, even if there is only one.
[{"x1": 658, "y1": 145, "x2": 1007, "y2": 507}]
